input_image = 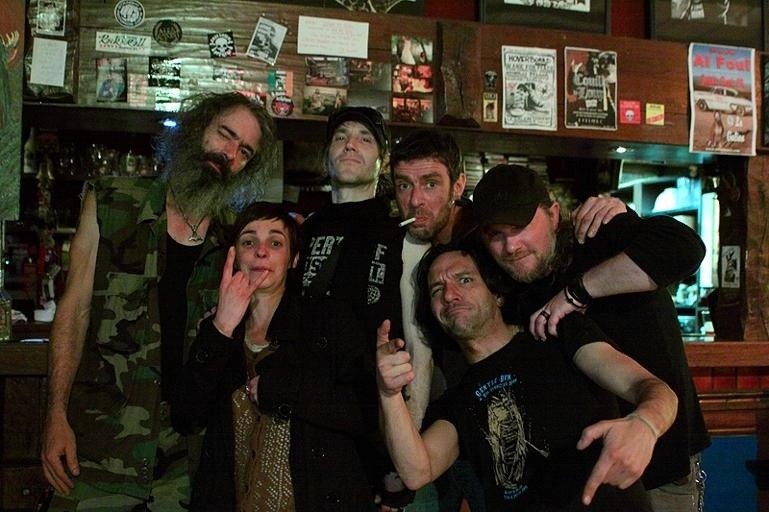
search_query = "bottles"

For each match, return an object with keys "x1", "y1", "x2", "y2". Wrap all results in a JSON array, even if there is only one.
[
  {"x1": 464, "y1": 164, "x2": 551, "y2": 237},
  {"x1": 326, "y1": 107, "x2": 391, "y2": 151}
]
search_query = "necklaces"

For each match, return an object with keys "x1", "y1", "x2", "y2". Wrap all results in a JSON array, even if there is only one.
[{"x1": 166, "y1": 181, "x2": 206, "y2": 242}]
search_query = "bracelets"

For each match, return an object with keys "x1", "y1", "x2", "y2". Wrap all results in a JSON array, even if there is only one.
[
  {"x1": 627, "y1": 412, "x2": 659, "y2": 437},
  {"x1": 564, "y1": 275, "x2": 593, "y2": 309}
]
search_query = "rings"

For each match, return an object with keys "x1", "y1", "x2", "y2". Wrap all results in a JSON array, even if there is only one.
[{"x1": 541, "y1": 311, "x2": 551, "y2": 320}]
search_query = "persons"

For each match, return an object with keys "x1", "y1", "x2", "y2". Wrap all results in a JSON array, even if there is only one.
[
  {"x1": 462, "y1": 164, "x2": 712, "y2": 506},
  {"x1": 37, "y1": 90, "x2": 403, "y2": 511},
  {"x1": 375, "y1": 240, "x2": 679, "y2": 507},
  {"x1": 333, "y1": 131, "x2": 639, "y2": 329}
]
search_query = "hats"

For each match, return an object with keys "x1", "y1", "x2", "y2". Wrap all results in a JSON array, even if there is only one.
[{"x1": 694, "y1": 86, "x2": 753, "y2": 116}]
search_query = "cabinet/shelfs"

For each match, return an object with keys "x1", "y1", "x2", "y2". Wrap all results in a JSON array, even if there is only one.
[
  {"x1": 21, "y1": 123, "x2": 155, "y2": 226},
  {"x1": 0, "y1": 262, "x2": 12, "y2": 343}
]
